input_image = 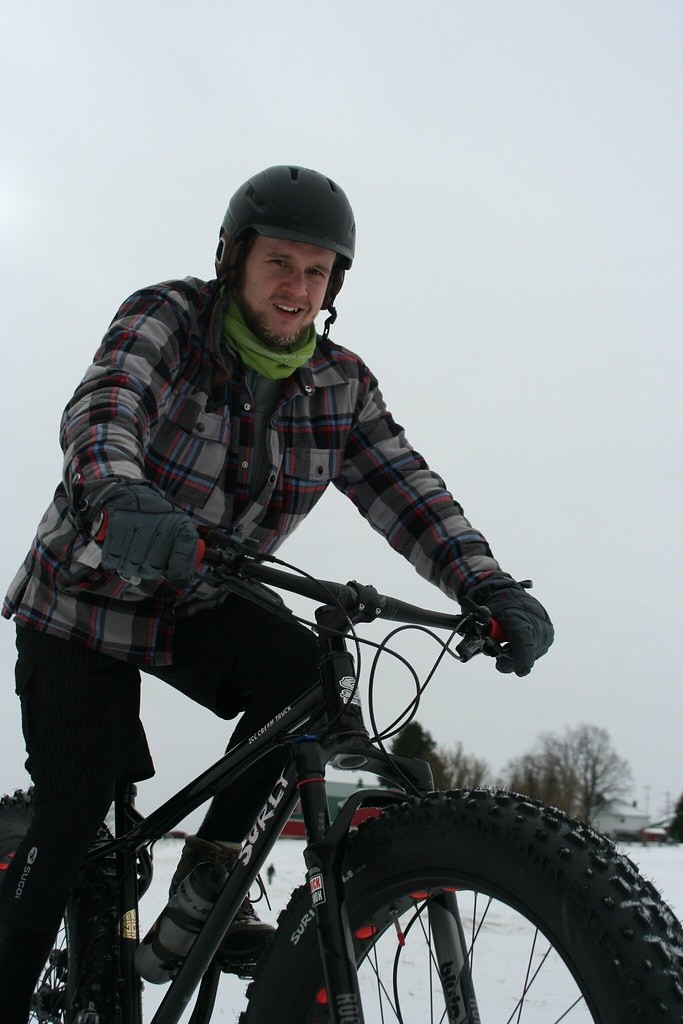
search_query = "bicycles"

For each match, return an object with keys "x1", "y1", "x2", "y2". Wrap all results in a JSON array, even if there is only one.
[{"x1": 0, "y1": 495, "x2": 683, "y2": 1024}]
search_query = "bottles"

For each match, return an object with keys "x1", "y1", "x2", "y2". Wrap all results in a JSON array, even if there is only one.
[{"x1": 135, "y1": 862, "x2": 230, "y2": 984}]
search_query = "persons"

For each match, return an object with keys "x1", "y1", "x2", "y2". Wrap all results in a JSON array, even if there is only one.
[
  {"x1": 268, "y1": 864, "x2": 275, "y2": 885},
  {"x1": 0, "y1": 165, "x2": 554, "y2": 1023}
]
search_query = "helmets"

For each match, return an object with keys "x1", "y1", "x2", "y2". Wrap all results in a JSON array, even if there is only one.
[{"x1": 215, "y1": 167, "x2": 356, "y2": 289}]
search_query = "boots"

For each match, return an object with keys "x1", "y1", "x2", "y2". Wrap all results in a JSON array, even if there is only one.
[{"x1": 168, "y1": 836, "x2": 276, "y2": 978}]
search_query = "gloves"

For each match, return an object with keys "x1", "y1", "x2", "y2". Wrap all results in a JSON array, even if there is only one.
[
  {"x1": 478, "y1": 580, "x2": 555, "y2": 677},
  {"x1": 97, "y1": 480, "x2": 201, "y2": 585}
]
